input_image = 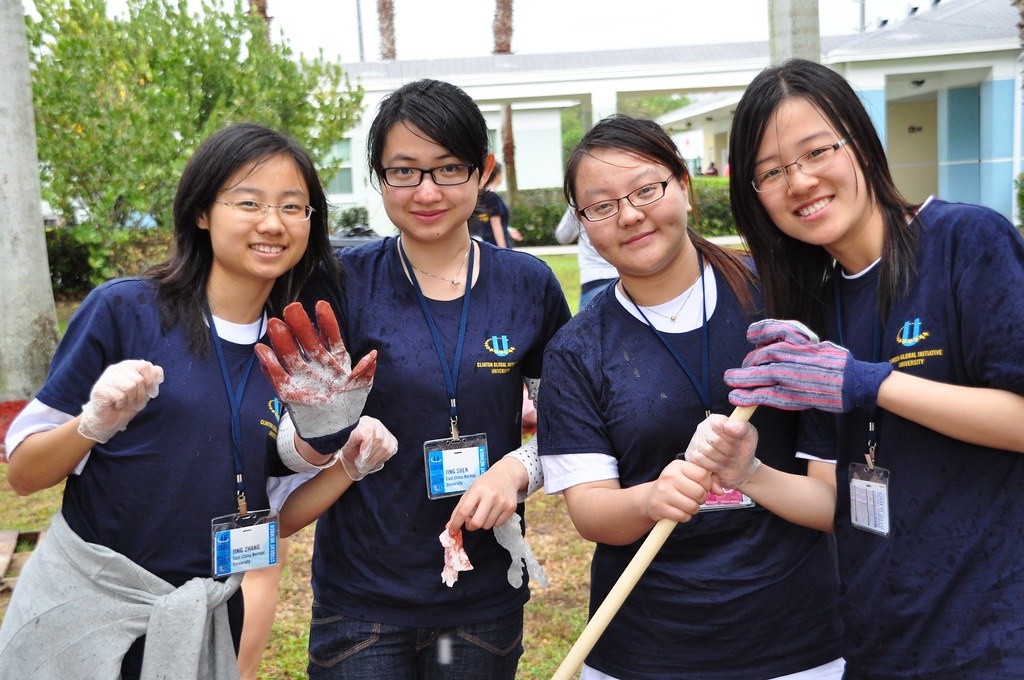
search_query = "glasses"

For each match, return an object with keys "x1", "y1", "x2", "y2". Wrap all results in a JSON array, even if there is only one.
[
  {"x1": 377, "y1": 164, "x2": 476, "y2": 187},
  {"x1": 575, "y1": 174, "x2": 672, "y2": 223},
  {"x1": 751, "y1": 134, "x2": 851, "y2": 195},
  {"x1": 216, "y1": 200, "x2": 316, "y2": 223}
]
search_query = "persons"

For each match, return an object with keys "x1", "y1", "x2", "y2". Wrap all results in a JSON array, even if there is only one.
[
  {"x1": 0, "y1": 122, "x2": 351, "y2": 679},
  {"x1": 251, "y1": 79, "x2": 573, "y2": 679},
  {"x1": 729, "y1": 59, "x2": 1024, "y2": 680},
  {"x1": 534, "y1": 113, "x2": 837, "y2": 680},
  {"x1": 474, "y1": 161, "x2": 526, "y2": 249}
]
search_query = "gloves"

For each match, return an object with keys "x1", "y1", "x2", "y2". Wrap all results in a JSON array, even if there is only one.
[
  {"x1": 724, "y1": 318, "x2": 892, "y2": 416},
  {"x1": 253, "y1": 299, "x2": 377, "y2": 456}
]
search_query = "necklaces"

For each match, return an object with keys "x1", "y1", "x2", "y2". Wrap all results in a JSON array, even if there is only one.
[{"x1": 636, "y1": 276, "x2": 700, "y2": 323}]
